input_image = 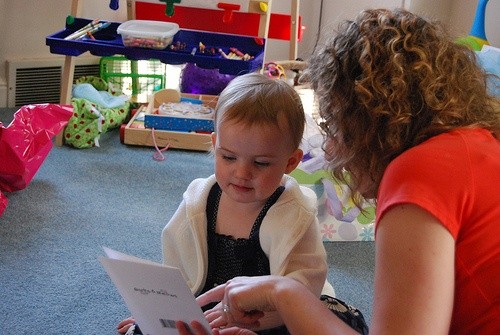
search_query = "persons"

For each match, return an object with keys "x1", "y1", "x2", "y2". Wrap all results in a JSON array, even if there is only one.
[
  {"x1": 115, "y1": 71, "x2": 329, "y2": 334},
  {"x1": 172, "y1": 9, "x2": 499, "y2": 335}
]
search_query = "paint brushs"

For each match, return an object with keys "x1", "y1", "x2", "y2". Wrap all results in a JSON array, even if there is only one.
[{"x1": 63, "y1": 17, "x2": 255, "y2": 62}]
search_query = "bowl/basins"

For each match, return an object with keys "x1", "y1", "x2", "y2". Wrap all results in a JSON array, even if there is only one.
[{"x1": 116, "y1": 20, "x2": 179, "y2": 49}]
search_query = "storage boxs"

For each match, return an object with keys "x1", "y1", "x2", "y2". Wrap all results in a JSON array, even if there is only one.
[{"x1": 46, "y1": 14, "x2": 267, "y2": 152}]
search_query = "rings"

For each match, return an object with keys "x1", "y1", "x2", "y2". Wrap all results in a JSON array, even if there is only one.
[{"x1": 221, "y1": 303, "x2": 231, "y2": 313}]
